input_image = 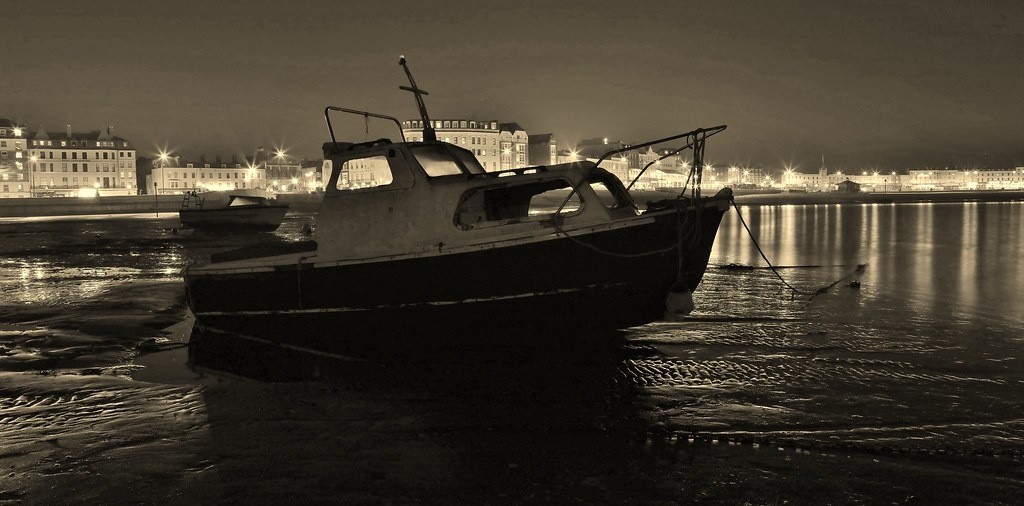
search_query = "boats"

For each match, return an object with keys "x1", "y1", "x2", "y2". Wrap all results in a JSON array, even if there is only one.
[
  {"x1": 161, "y1": 51, "x2": 739, "y2": 334},
  {"x1": 174, "y1": 188, "x2": 289, "y2": 228}
]
